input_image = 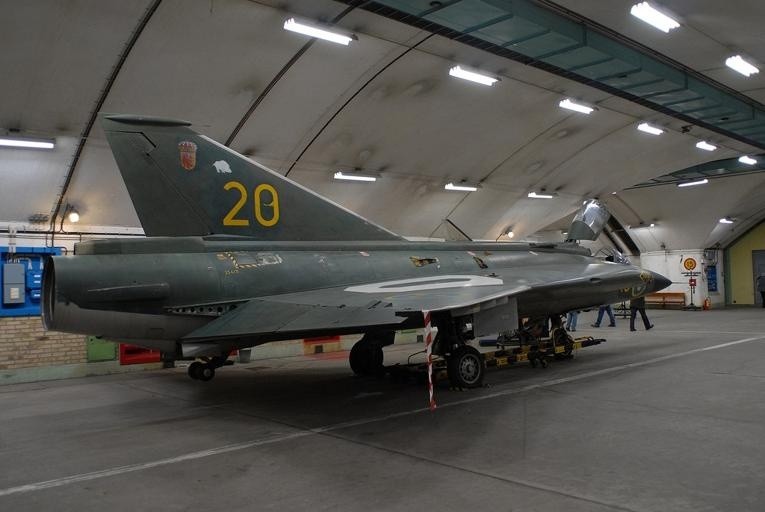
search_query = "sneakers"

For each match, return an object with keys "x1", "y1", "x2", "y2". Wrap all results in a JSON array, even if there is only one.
[
  {"x1": 590, "y1": 323, "x2": 616, "y2": 327},
  {"x1": 566, "y1": 327, "x2": 577, "y2": 331},
  {"x1": 629, "y1": 324, "x2": 654, "y2": 331}
]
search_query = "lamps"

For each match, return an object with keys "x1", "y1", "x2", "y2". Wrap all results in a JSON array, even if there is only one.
[
  {"x1": 630, "y1": 1, "x2": 683, "y2": 34},
  {"x1": 719, "y1": 216, "x2": 738, "y2": 223},
  {"x1": 281, "y1": 16, "x2": 358, "y2": 47},
  {"x1": 334, "y1": 171, "x2": 383, "y2": 183},
  {"x1": 69, "y1": 205, "x2": 79, "y2": 222},
  {"x1": 677, "y1": 179, "x2": 708, "y2": 188},
  {"x1": 1, "y1": 135, "x2": 56, "y2": 151},
  {"x1": 448, "y1": 64, "x2": 502, "y2": 86},
  {"x1": 695, "y1": 139, "x2": 722, "y2": 151},
  {"x1": 527, "y1": 191, "x2": 560, "y2": 199},
  {"x1": 633, "y1": 120, "x2": 669, "y2": 137},
  {"x1": 737, "y1": 154, "x2": 759, "y2": 165},
  {"x1": 559, "y1": 96, "x2": 600, "y2": 115},
  {"x1": 726, "y1": 52, "x2": 760, "y2": 79},
  {"x1": 444, "y1": 182, "x2": 484, "y2": 193}
]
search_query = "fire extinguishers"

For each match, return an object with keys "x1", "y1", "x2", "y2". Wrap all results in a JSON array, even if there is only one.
[{"x1": 704, "y1": 299, "x2": 710, "y2": 309}]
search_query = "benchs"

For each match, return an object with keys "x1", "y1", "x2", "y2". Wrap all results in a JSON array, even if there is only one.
[{"x1": 645, "y1": 293, "x2": 685, "y2": 310}]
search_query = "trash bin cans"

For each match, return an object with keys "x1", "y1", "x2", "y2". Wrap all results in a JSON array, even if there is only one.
[{"x1": 238, "y1": 348, "x2": 251, "y2": 364}]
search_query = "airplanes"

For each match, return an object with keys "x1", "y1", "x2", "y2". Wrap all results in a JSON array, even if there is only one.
[{"x1": 41, "y1": 113, "x2": 673, "y2": 389}]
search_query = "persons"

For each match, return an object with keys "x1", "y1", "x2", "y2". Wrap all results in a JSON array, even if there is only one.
[
  {"x1": 564, "y1": 311, "x2": 578, "y2": 332},
  {"x1": 628, "y1": 295, "x2": 655, "y2": 331},
  {"x1": 590, "y1": 304, "x2": 616, "y2": 327}
]
